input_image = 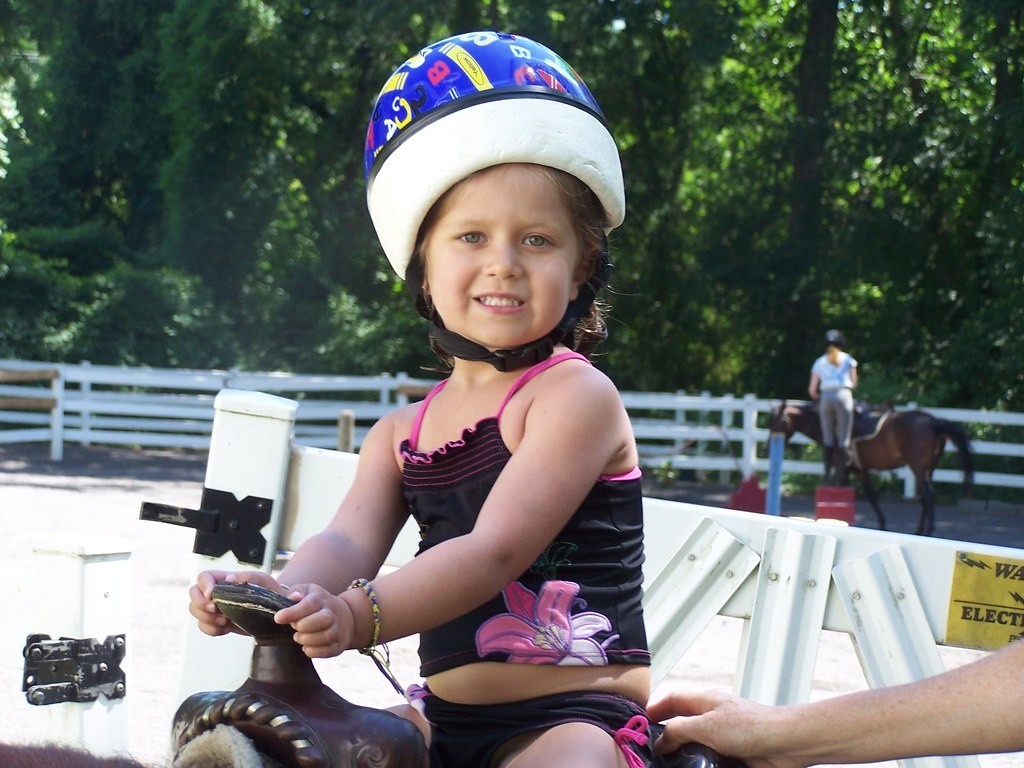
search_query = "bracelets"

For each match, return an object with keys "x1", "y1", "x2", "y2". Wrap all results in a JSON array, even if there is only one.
[
  {"x1": 347, "y1": 578, "x2": 410, "y2": 702},
  {"x1": 280, "y1": 583, "x2": 289, "y2": 590}
]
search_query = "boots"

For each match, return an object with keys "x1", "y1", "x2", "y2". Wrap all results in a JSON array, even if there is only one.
[
  {"x1": 836, "y1": 446, "x2": 852, "y2": 486},
  {"x1": 821, "y1": 444, "x2": 834, "y2": 483}
]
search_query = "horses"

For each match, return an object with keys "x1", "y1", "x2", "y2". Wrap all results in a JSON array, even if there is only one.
[{"x1": 767, "y1": 399, "x2": 975, "y2": 538}]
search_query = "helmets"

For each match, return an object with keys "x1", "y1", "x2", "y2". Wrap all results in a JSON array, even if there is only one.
[
  {"x1": 364, "y1": 31, "x2": 625, "y2": 278},
  {"x1": 824, "y1": 330, "x2": 845, "y2": 348}
]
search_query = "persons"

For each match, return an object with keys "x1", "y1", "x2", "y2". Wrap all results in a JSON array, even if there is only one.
[
  {"x1": 646, "y1": 636, "x2": 1024, "y2": 767},
  {"x1": 187, "y1": 30, "x2": 652, "y2": 768},
  {"x1": 809, "y1": 330, "x2": 858, "y2": 487}
]
